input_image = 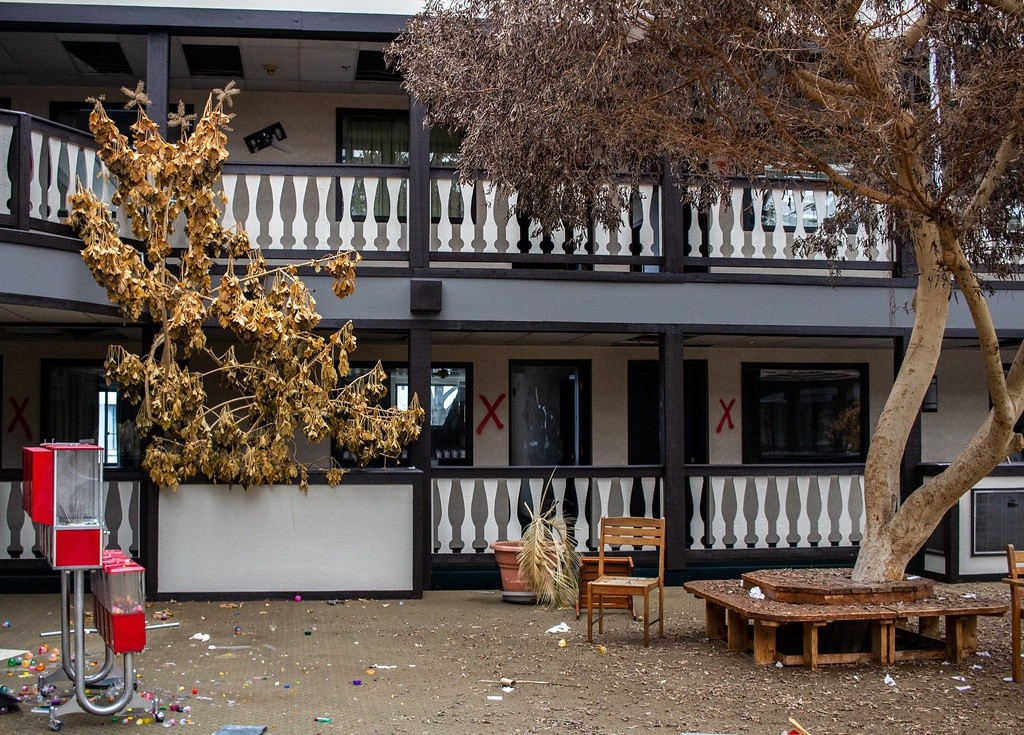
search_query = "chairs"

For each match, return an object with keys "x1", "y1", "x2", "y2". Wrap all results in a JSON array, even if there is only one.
[
  {"x1": 587, "y1": 517, "x2": 667, "y2": 648},
  {"x1": 1003, "y1": 544, "x2": 1024, "y2": 683},
  {"x1": 575, "y1": 554, "x2": 638, "y2": 621}
]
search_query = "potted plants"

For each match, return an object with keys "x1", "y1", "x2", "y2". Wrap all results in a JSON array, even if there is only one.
[{"x1": 489, "y1": 465, "x2": 579, "y2": 607}]
